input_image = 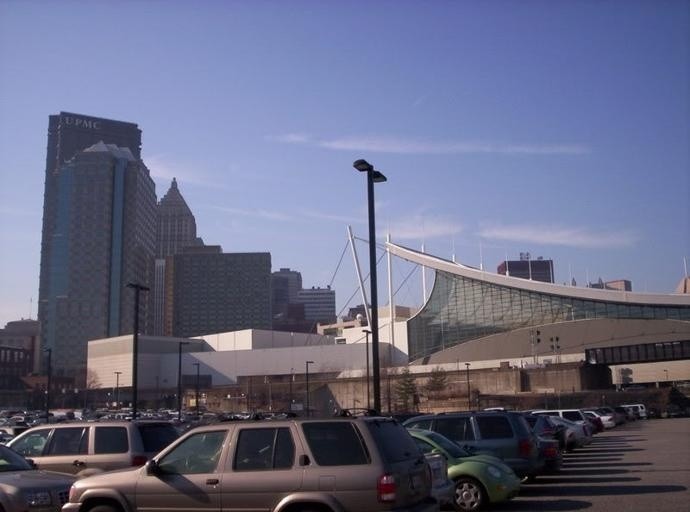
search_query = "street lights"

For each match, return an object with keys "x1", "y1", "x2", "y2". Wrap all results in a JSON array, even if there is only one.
[
  {"x1": 126, "y1": 282, "x2": 149, "y2": 421},
  {"x1": 354, "y1": 160, "x2": 387, "y2": 413},
  {"x1": 362, "y1": 329, "x2": 371, "y2": 412},
  {"x1": 179, "y1": 342, "x2": 188, "y2": 421},
  {"x1": 44, "y1": 348, "x2": 52, "y2": 424},
  {"x1": 114, "y1": 372, "x2": 121, "y2": 409},
  {"x1": 464, "y1": 363, "x2": 470, "y2": 412},
  {"x1": 248, "y1": 361, "x2": 314, "y2": 418},
  {"x1": 193, "y1": 360, "x2": 199, "y2": 417}
]
